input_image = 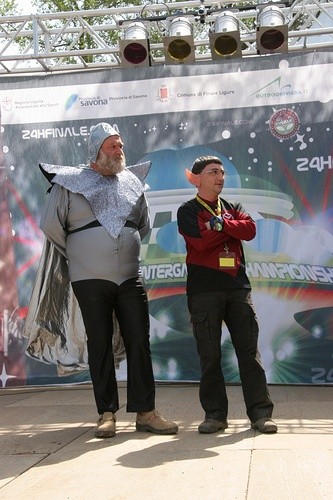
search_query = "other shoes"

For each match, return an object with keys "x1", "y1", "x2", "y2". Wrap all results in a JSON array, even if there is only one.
[
  {"x1": 136, "y1": 408, "x2": 179, "y2": 435},
  {"x1": 251, "y1": 416, "x2": 278, "y2": 434},
  {"x1": 199, "y1": 415, "x2": 229, "y2": 435},
  {"x1": 94, "y1": 411, "x2": 118, "y2": 439}
]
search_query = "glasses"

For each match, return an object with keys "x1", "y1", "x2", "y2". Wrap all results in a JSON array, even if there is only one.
[{"x1": 200, "y1": 168, "x2": 228, "y2": 177}]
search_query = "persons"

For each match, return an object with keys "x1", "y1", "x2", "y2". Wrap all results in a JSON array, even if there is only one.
[
  {"x1": 177, "y1": 155, "x2": 277, "y2": 433},
  {"x1": 39, "y1": 123, "x2": 178, "y2": 438}
]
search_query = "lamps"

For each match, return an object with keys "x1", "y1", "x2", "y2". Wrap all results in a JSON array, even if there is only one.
[
  {"x1": 162, "y1": 18, "x2": 197, "y2": 65},
  {"x1": 255, "y1": 4, "x2": 288, "y2": 55},
  {"x1": 208, "y1": 13, "x2": 241, "y2": 63},
  {"x1": 123, "y1": 22, "x2": 151, "y2": 66}
]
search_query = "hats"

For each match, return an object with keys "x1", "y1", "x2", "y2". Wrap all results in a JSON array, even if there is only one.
[{"x1": 87, "y1": 123, "x2": 120, "y2": 163}]
